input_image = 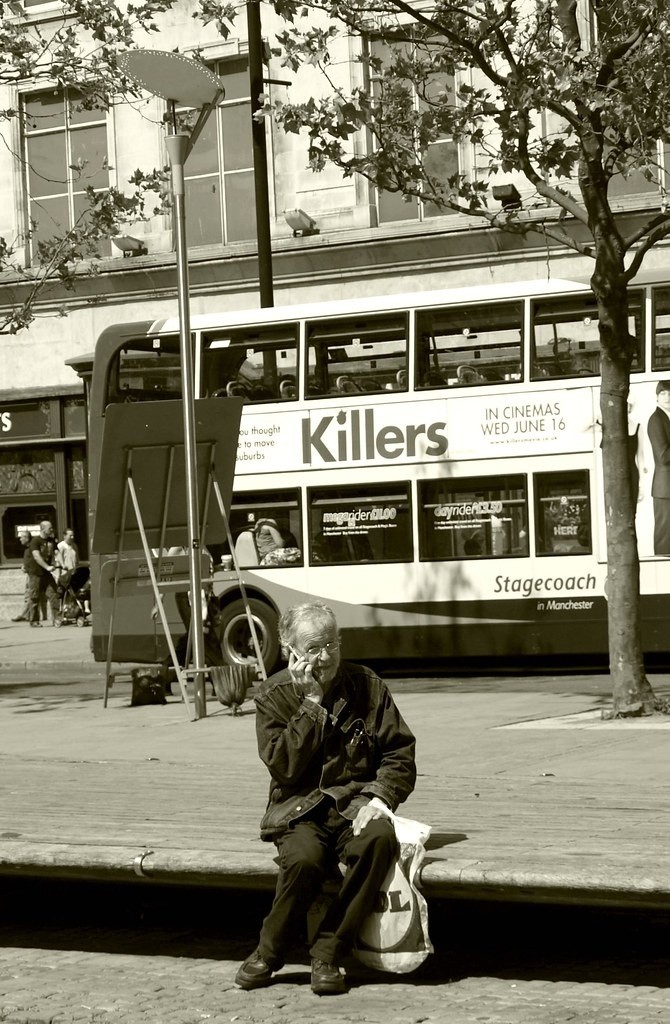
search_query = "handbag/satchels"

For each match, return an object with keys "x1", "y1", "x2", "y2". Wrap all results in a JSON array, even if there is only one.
[
  {"x1": 258, "y1": 548, "x2": 304, "y2": 566},
  {"x1": 345, "y1": 799, "x2": 434, "y2": 974}
]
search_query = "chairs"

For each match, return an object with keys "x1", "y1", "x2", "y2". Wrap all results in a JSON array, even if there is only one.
[
  {"x1": 234, "y1": 529, "x2": 259, "y2": 567},
  {"x1": 211, "y1": 365, "x2": 481, "y2": 401}
]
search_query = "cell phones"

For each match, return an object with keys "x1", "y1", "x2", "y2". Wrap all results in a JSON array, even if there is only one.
[{"x1": 287, "y1": 646, "x2": 319, "y2": 682}]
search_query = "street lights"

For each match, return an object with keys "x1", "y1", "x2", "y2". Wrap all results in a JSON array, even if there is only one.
[{"x1": 116, "y1": 50, "x2": 225, "y2": 720}]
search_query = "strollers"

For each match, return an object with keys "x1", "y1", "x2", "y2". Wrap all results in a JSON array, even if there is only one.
[{"x1": 47, "y1": 566, "x2": 93, "y2": 627}]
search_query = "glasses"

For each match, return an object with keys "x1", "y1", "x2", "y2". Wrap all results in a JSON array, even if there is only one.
[{"x1": 291, "y1": 642, "x2": 339, "y2": 654}]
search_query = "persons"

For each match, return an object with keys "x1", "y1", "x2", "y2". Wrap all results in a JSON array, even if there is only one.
[
  {"x1": 10, "y1": 521, "x2": 80, "y2": 627},
  {"x1": 235, "y1": 602, "x2": 417, "y2": 993},
  {"x1": 253, "y1": 509, "x2": 293, "y2": 560},
  {"x1": 646, "y1": 381, "x2": 670, "y2": 558}
]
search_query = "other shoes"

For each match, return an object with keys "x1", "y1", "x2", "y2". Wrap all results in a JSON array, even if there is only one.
[
  {"x1": 30, "y1": 622, "x2": 43, "y2": 627},
  {"x1": 11, "y1": 616, "x2": 29, "y2": 622}
]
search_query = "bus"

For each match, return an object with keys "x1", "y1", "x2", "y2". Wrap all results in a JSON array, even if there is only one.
[{"x1": 88, "y1": 265, "x2": 670, "y2": 684}]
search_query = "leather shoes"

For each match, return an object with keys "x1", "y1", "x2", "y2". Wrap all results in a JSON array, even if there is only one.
[
  {"x1": 235, "y1": 945, "x2": 285, "y2": 986},
  {"x1": 310, "y1": 958, "x2": 343, "y2": 993}
]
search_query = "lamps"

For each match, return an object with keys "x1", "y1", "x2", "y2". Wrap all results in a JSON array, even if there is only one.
[
  {"x1": 108, "y1": 234, "x2": 145, "y2": 257},
  {"x1": 493, "y1": 183, "x2": 523, "y2": 211},
  {"x1": 282, "y1": 208, "x2": 317, "y2": 237}
]
